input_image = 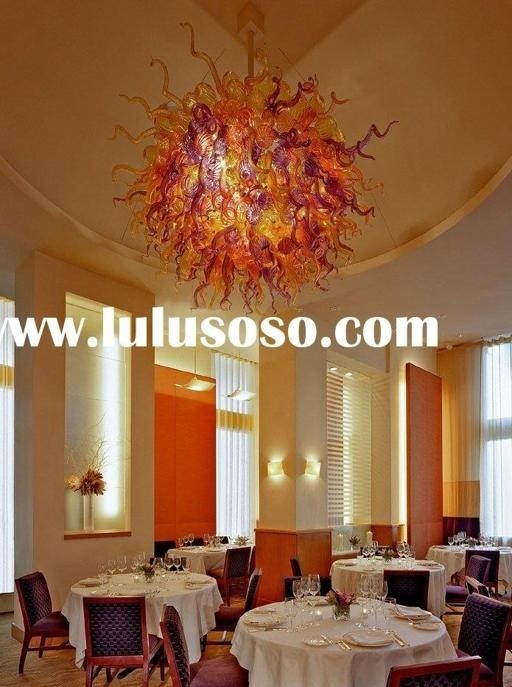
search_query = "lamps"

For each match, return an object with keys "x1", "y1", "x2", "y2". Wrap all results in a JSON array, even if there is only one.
[
  {"x1": 226, "y1": 342, "x2": 257, "y2": 401},
  {"x1": 303, "y1": 460, "x2": 322, "y2": 475},
  {"x1": 176, "y1": 300, "x2": 218, "y2": 392},
  {"x1": 104, "y1": 0, "x2": 400, "y2": 313},
  {"x1": 267, "y1": 460, "x2": 283, "y2": 477}
]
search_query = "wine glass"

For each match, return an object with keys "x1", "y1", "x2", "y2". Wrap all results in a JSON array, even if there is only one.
[
  {"x1": 448, "y1": 531, "x2": 494, "y2": 552},
  {"x1": 176, "y1": 530, "x2": 251, "y2": 549},
  {"x1": 96, "y1": 550, "x2": 191, "y2": 591},
  {"x1": 355, "y1": 575, "x2": 397, "y2": 634},
  {"x1": 361, "y1": 540, "x2": 411, "y2": 569},
  {"x1": 284, "y1": 574, "x2": 321, "y2": 632}
]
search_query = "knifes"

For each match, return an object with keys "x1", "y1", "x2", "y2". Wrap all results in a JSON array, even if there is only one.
[
  {"x1": 389, "y1": 630, "x2": 410, "y2": 647},
  {"x1": 320, "y1": 633, "x2": 351, "y2": 651}
]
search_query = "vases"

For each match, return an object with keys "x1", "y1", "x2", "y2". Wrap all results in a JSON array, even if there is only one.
[{"x1": 81, "y1": 495, "x2": 94, "y2": 534}]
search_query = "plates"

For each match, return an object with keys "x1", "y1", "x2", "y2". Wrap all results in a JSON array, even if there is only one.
[
  {"x1": 345, "y1": 629, "x2": 392, "y2": 648},
  {"x1": 301, "y1": 635, "x2": 331, "y2": 647},
  {"x1": 395, "y1": 609, "x2": 441, "y2": 631},
  {"x1": 180, "y1": 546, "x2": 228, "y2": 553},
  {"x1": 242, "y1": 612, "x2": 284, "y2": 626},
  {"x1": 77, "y1": 574, "x2": 212, "y2": 595}
]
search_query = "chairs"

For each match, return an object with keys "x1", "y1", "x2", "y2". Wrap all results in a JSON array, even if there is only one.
[{"x1": 14, "y1": 541, "x2": 512, "y2": 686}]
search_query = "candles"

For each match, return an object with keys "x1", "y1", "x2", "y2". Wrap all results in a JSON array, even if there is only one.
[
  {"x1": 336, "y1": 533, "x2": 344, "y2": 548},
  {"x1": 366, "y1": 530, "x2": 373, "y2": 545}
]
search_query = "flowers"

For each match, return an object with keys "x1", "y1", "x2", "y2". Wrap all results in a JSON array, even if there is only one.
[{"x1": 63, "y1": 411, "x2": 113, "y2": 496}]
